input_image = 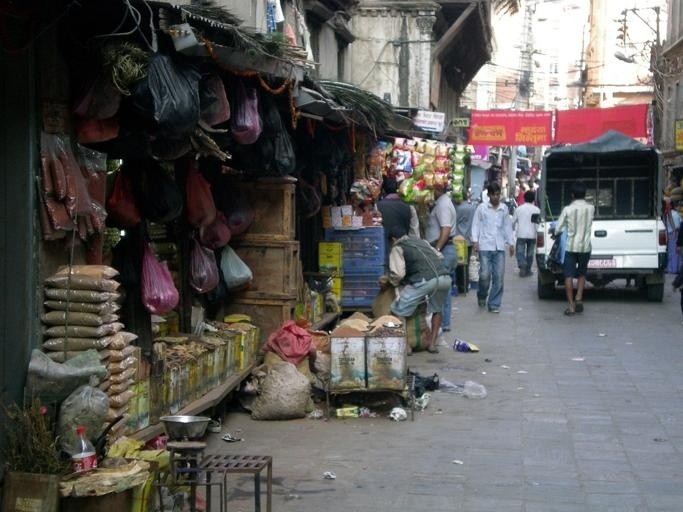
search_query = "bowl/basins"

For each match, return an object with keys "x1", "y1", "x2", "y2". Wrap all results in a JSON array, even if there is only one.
[{"x1": 158, "y1": 415, "x2": 215, "y2": 441}]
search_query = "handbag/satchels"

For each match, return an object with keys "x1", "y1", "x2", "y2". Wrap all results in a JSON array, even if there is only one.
[{"x1": 468, "y1": 247, "x2": 480, "y2": 284}]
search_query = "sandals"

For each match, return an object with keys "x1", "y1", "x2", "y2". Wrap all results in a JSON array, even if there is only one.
[{"x1": 564, "y1": 300, "x2": 584, "y2": 316}]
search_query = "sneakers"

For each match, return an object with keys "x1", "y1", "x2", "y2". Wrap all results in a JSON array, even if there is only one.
[
  {"x1": 519, "y1": 271, "x2": 533, "y2": 278},
  {"x1": 477, "y1": 291, "x2": 501, "y2": 313}
]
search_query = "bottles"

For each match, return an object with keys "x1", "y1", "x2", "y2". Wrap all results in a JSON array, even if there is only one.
[{"x1": 72, "y1": 427, "x2": 97, "y2": 473}]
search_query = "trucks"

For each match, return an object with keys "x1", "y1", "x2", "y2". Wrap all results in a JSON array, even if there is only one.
[{"x1": 529, "y1": 144, "x2": 671, "y2": 300}]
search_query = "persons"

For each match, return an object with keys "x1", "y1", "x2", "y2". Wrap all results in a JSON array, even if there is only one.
[
  {"x1": 672, "y1": 222, "x2": 683, "y2": 312},
  {"x1": 553, "y1": 182, "x2": 595, "y2": 315},
  {"x1": 664, "y1": 202, "x2": 683, "y2": 273},
  {"x1": 374, "y1": 176, "x2": 540, "y2": 353}
]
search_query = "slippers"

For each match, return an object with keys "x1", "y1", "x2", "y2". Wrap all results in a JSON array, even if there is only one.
[{"x1": 427, "y1": 345, "x2": 440, "y2": 354}]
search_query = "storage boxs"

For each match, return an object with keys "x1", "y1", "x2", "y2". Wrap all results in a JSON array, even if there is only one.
[
  {"x1": 129, "y1": 322, "x2": 229, "y2": 438},
  {"x1": 229, "y1": 181, "x2": 409, "y2": 393},
  {"x1": 454, "y1": 238, "x2": 469, "y2": 264}
]
